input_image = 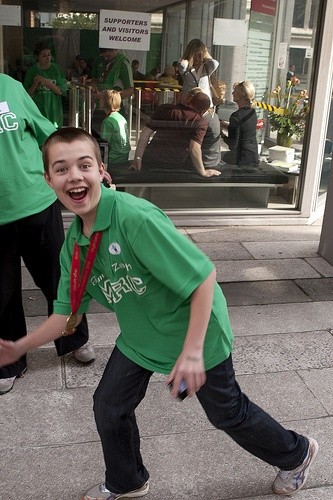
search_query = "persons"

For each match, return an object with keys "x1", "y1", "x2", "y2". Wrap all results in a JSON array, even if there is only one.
[
  {"x1": 99, "y1": 89, "x2": 134, "y2": 182},
  {"x1": 131, "y1": 60, "x2": 179, "y2": 88},
  {"x1": 69, "y1": 54, "x2": 111, "y2": 79},
  {"x1": 25, "y1": 42, "x2": 68, "y2": 128},
  {"x1": 128, "y1": 88, "x2": 222, "y2": 177},
  {"x1": 176, "y1": 38, "x2": 219, "y2": 109},
  {"x1": 221, "y1": 80, "x2": 259, "y2": 166},
  {"x1": 0, "y1": 72, "x2": 111, "y2": 394},
  {"x1": 284, "y1": 64, "x2": 295, "y2": 102},
  {"x1": 0, "y1": 127, "x2": 319, "y2": 500}
]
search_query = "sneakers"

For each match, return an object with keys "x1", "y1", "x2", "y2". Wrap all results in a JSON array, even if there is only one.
[
  {"x1": 84, "y1": 480, "x2": 149, "y2": 500},
  {"x1": 74, "y1": 343, "x2": 95, "y2": 363},
  {"x1": 272, "y1": 437, "x2": 319, "y2": 495},
  {"x1": 0, "y1": 368, "x2": 27, "y2": 391}
]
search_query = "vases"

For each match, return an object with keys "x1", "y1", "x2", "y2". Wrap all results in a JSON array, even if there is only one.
[{"x1": 277, "y1": 132, "x2": 294, "y2": 147}]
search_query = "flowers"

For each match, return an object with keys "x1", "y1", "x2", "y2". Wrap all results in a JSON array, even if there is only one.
[{"x1": 268, "y1": 76, "x2": 309, "y2": 137}]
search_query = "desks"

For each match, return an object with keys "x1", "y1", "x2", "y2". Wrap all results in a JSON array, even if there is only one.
[{"x1": 132, "y1": 80, "x2": 162, "y2": 87}]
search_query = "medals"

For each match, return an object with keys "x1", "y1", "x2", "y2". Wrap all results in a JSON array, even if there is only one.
[{"x1": 62, "y1": 314, "x2": 77, "y2": 336}]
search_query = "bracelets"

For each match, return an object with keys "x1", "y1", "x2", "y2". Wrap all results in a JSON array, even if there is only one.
[{"x1": 135, "y1": 156, "x2": 142, "y2": 160}]
